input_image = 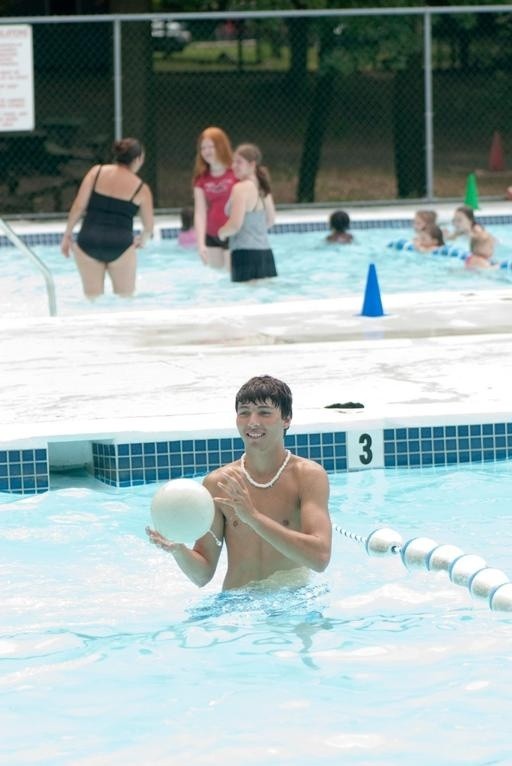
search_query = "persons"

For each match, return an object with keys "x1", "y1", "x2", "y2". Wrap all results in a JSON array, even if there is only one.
[
  {"x1": 411, "y1": 222, "x2": 446, "y2": 252},
  {"x1": 463, "y1": 231, "x2": 507, "y2": 270},
  {"x1": 63, "y1": 137, "x2": 155, "y2": 298},
  {"x1": 145, "y1": 374, "x2": 335, "y2": 594},
  {"x1": 191, "y1": 126, "x2": 240, "y2": 273},
  {"x1": 217, "y1": 142, "x2": 280, "y2": 282},
  {"x1": 177, "y1": 206, "x2": 197, "y2": 246},
  {"x1": 413, "y1": 207, "x2": 437, "y2": 231},
  {"x1": 327, "y1": 209, "x2": 353, "y2": 243},
  {"x1": 444, "y1": 206, "x2": 484, "y2": 240}
]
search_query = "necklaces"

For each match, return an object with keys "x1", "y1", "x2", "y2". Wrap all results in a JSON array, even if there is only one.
[{"x1": 240, "y1": 449, "x2": 294, "y2": 488}]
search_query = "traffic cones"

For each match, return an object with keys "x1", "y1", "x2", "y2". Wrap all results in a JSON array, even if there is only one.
[
  {"x1": 355, "y1": 264, "x2": 387, "y2": 316},
  {"x1": 464, "y1": 174, "x2": 480, "y2": 209},
  {"x1": 490, "y1": 131, "x2": 504, "y2": 171}
]
